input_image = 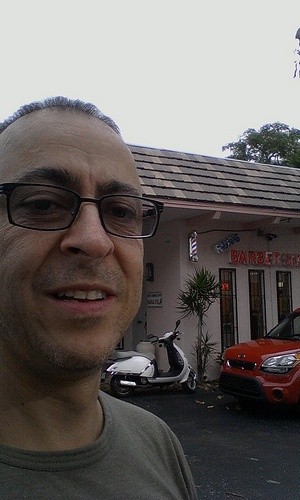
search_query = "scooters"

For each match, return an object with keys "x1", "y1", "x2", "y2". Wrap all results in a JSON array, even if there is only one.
[{"x1": 106, "y1": 319, "x2": 198, "y2": 398}]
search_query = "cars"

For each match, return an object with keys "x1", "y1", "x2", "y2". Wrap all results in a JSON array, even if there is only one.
[{"x1": 218, "y1": 307, "x2": 300, "y2": 413}]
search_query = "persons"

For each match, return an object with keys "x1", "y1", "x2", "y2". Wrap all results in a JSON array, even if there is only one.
[{"x1": 0, "y1": 97, "x2": 197, "y2": 500}]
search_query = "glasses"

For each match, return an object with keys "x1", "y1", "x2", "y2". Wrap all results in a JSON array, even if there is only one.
[{"x1": 0, "y1": 182, "x2": 164, "y2": 240}]
